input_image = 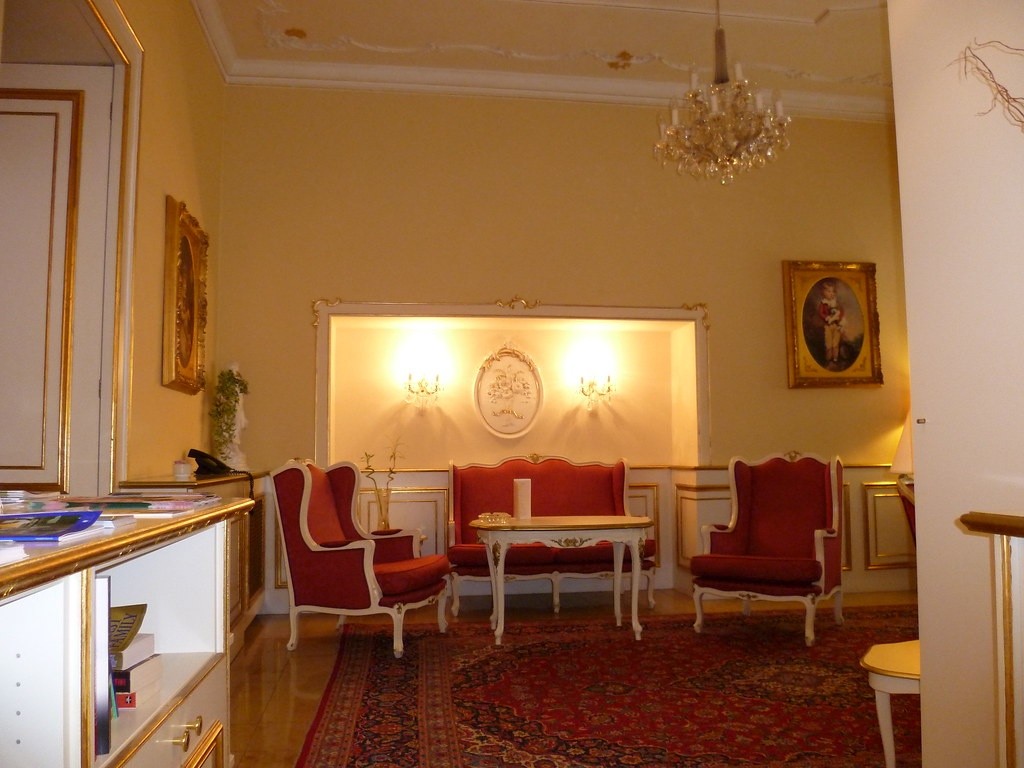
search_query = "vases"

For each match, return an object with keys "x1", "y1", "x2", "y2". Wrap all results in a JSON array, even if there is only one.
[{"x1": 174, "y1": 463, "x2": 193, "y2": 481}]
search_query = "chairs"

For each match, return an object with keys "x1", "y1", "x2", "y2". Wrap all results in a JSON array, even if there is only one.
[
  {"x1": 897, "y1": 473, "x2": 915, "y2": 546},
  {"x1": 862, "y1": 638, "x2": 921, "y2": 768},
  {"x1": 690, "y1": 455, "x2": 851, "y2": 645},
  {"x1": 270, "y1": 458, "x2": 452, "y2": 657}
]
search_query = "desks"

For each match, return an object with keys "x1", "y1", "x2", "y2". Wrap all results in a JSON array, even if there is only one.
[{"x1": 469, "y1": 516, "x2": 656, "y2": 645}]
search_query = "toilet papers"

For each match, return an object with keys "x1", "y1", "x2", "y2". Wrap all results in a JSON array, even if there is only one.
[{"x1": 513, "y1": 478, "x2": 531, "y2": 517}]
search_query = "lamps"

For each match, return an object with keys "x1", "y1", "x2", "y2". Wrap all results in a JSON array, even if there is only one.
[
  {"x1": 579, "y1": 376, "x2": 615, "y2": 410},
  {"x1": 405, "y1": 373, "x2": 441, "y2": 409},
  {"x1": 653, "y1": 0, "x2": 791, "y2": 183}
]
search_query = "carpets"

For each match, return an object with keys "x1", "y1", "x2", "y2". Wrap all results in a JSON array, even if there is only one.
[{"x1": 298, "y1": 604, "x2": 920, "y2": 768}]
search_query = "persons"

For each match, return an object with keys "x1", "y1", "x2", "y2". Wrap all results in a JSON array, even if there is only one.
[{"x1": 817, "y1": 281, "x2": 846, "y2": 369}]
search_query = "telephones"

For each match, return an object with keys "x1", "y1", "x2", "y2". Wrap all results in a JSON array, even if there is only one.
[{"x1": 187, "y1": 448, "x2": 235, "y2": 475}]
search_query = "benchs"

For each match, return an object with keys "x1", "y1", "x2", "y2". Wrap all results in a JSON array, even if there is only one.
[{"x1": 448, "y1": 453, "x2": 659, "y2": 616}]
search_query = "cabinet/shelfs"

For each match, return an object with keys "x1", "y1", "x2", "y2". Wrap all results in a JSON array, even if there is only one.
[
  {"x1": 0, "y1": 503, "x2": 253, "y2": 768},
  {"x1": 188, "y1": 469, "x2": 270, "y2": 663}
]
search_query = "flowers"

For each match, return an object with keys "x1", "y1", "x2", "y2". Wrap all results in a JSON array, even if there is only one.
[{"x1": 176, "y1": 448, "x2": 190, "y2": 463}]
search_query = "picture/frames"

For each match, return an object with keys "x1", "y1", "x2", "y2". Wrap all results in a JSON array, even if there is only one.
[
  {"x1": 783, "y1": 260, "x2": 884, "y2": 390},
  {"x1": 166, "y1": 195, "x2": 211, "y2": 395}
]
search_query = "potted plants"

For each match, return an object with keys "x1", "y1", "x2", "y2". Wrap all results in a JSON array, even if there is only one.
[{"x1": 360, "y1": 437, "x2": 408, "y2": 530}]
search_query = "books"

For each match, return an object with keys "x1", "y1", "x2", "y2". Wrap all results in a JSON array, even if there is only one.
[
  {"x1": 0, "y1": 489, "x2": 222, "y2": 565},
  {"x1": 95, "y1": 575, "x2": 164, "y2": 756}
]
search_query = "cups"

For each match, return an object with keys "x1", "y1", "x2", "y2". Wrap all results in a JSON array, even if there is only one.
[{"x1": 172, "y1": 464, "x2": 192, "y2": 476}]
